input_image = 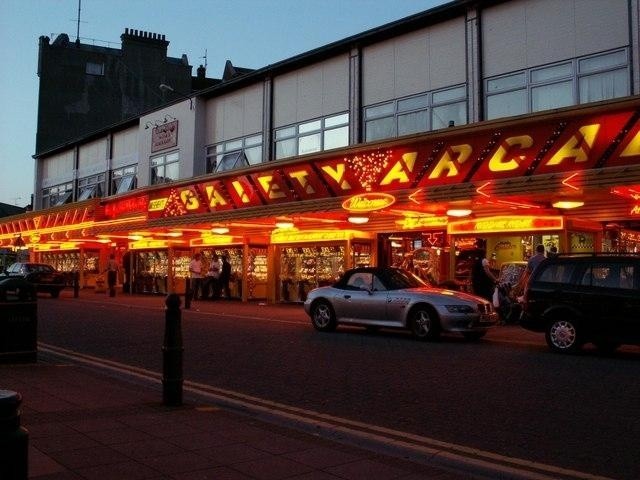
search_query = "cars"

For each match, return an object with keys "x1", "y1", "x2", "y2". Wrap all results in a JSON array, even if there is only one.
[
  {"x1": 0, "y1": 262, "x2": 66, "y2": 299},
  {"x1": 301, "y1": 265, "x2": 501, "y2": 342}
]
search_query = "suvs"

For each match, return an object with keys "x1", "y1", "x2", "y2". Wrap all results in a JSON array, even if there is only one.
[{"x1": 518, "y1": 247, "x2": 640, "y2": 355}]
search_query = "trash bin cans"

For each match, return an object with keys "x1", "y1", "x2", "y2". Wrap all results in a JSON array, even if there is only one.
[
  {"x1": 282, "y1": 277, "x2": 291, "y2": 303},
  {"x1": 1, "y1": 276, "x2": 38, "y2": 365},
  {"x1": 297, "y1": 280, "x2": 307, "y2": 303}
]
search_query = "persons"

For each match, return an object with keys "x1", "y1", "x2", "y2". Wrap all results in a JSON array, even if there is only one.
[
  {"x1": 469, "y1": 247, "x2": 499, "y2": 307},
  {"x1": 203, "y1": 253, "x2": 220, "y2": 300},
  {"x1": 107, "y1": 252, "x2": 120, "y2": 297},
  {"x1": 510, "y1": 242, "x2": 547, "y2": 297},
  {"x1": 188, "y1": 253, "x2": 204, "y2": 300},
  {"x1": 214, "y1": 254, "x2": 232, "y2": 302}
]
